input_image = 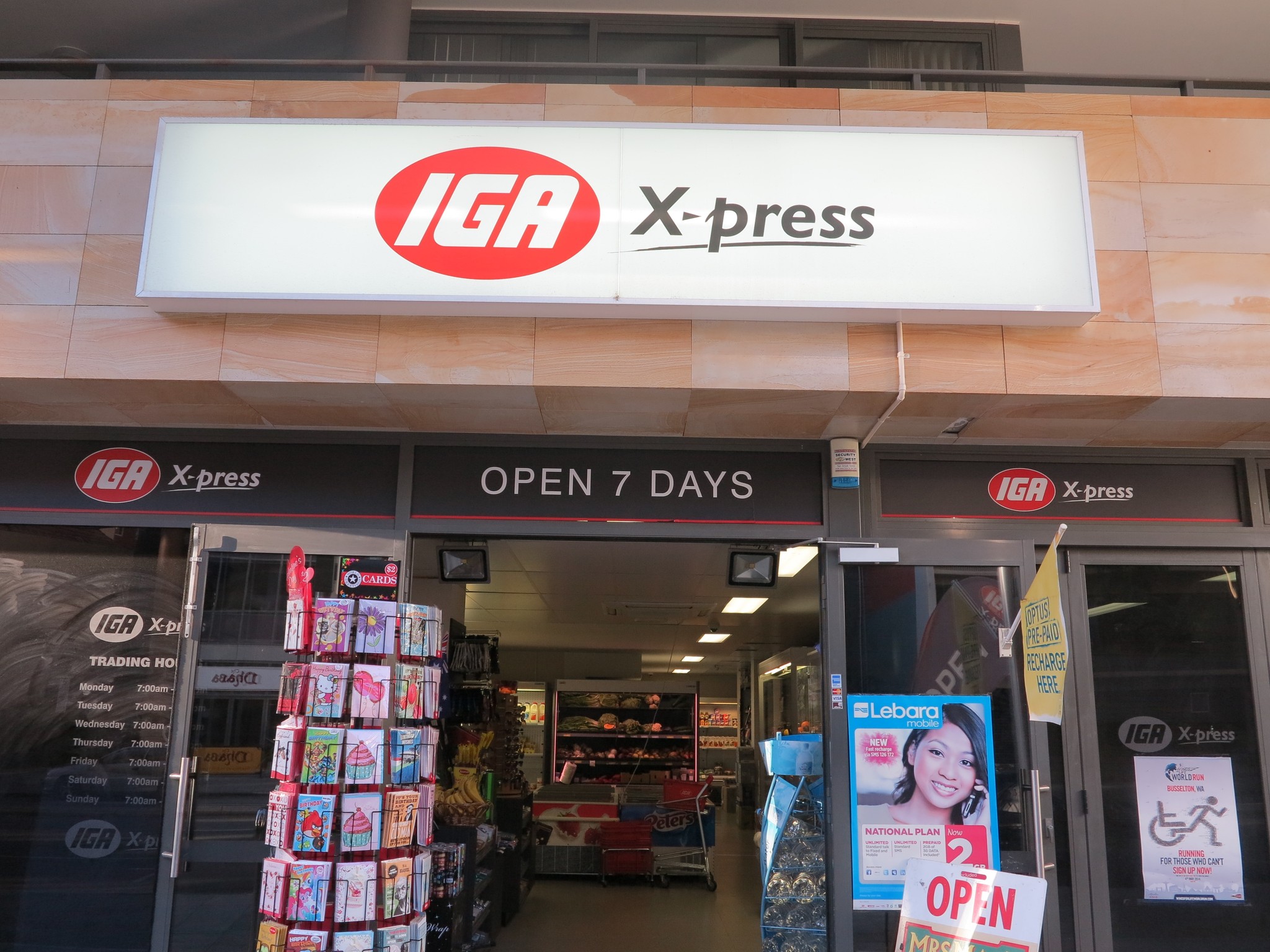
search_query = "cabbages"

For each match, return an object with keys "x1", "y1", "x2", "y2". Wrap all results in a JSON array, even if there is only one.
[{"x1": 581, "y1": 743, "x2": 618, "y2": 759}]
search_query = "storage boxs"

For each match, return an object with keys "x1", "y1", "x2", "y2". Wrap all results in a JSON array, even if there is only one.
[
  {"x1": 620, "y1": 768, "x2": 695, "y2": 784},
  {"x1": 721, "y1": 745, "x2": 755, "y2": 829}
]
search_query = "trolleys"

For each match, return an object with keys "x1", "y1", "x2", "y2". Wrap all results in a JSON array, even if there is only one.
[{"x1": 652, "y1": 775, "x2": 718, "y2": 892}]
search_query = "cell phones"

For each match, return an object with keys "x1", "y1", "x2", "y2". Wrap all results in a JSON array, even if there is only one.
[{"x1": 961, "y1": 789, "x2": 983, "y2": 819}]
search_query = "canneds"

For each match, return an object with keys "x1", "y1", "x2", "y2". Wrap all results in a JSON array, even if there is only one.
[{"x1": 428, "y1": 842, "x2": 467, "y2": 898}]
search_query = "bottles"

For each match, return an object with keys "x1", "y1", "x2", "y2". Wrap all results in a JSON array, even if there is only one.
[
  {"x1": 796, "y1": 743, "x2": 813, "y2": 775},
  {"x1": 786, "y1": 902, "x2": 826, "y2": 930},
  {"x1": 517, "y1": 702, "x2": 545, "y2": 723},
  {"x1": 704, "y1": 711, "x2": 709, "y2": 726},
  {"x1": 762, "y1": 931, "x2": 827, "y2": 952},
  {"x1": 757, "y1": 807, "x2": 808, "y2": 844},
  {"x1": 763, "y1": 903, "x2": 798, "y2": 927},
  {"x1": 700, "y1": 712, "x2": 705, "y2": 726},
  {"x1": 767, "y1": 872, "x2": 826, "y2": 904},
  {"x1": 773, "y1": 827, "x2": 826, "y2": 875}
]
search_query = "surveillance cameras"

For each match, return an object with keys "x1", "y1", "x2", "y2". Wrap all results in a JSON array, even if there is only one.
[{"x1": 710, "y1": 628, "x2": 719, "y2": 633}]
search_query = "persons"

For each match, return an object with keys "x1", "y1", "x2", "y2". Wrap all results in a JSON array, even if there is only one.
[{"x1": 857, "y1": 703, "x2": 993, "y2": 884}]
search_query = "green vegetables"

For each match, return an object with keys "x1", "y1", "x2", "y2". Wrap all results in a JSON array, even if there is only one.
[
  {"x1": 597, "y1": 713, "x2": 651, "y2": 734},
  {"x1": 599, "y1": 694, "x2": 644, "y2": 709}
]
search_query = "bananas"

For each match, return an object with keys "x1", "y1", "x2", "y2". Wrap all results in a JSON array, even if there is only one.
[{"x1": 434, "y1": 773, "x2": 485, "y2": 818}]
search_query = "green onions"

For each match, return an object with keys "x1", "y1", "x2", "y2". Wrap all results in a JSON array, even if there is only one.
[
  {"x1": 557, "y1": 716, "x2": 598, "y2": 730},
  {"x1": 558, "y1": 692, "x2": 601, "y2": 707}
]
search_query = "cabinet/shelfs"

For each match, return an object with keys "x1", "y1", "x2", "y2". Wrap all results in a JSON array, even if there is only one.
[
  {"x1": 426, "y1": 792, "x2": 536, "y2": 952},
  {"x1": 554, "y1": 692, "x2": 696, "y2": 782},
  {"x1": 760, "y1": 776, "x2": 828, "y2": 942}
]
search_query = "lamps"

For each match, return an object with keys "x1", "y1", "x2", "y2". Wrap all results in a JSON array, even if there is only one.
[
  {"x1": 724, "y1": 543, "x2": 781, "y2": 590},
  {"x1": 435, "y1": 538, "x2": 492, "y2": 585}
]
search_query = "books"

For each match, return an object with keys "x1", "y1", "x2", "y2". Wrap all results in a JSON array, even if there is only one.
[
  {"x1": 264, "y1": 724, "x2": 439, "y2": 852},
  {"x1": 276, "y1": 598, "x2": 442, "y2": 718},
  {"x1": 256, "y1": 846, "x2": 432, "y2": 952}
]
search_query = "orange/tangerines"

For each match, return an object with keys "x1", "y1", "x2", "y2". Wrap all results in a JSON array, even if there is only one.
[
  {"x1": 628, "y1": 747, "x2": 659, "y2": 759},
  {"x1": 798, "y1": 721, "x2": 810, "y2": 733}
]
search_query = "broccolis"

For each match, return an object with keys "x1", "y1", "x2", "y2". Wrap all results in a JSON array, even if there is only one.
[
  {"x1": 572, "y1": 750, "x2": 585, "y2": 758},
  {"x1": 650, "y1": 723, "x2": 662, "y2": 732},
  {"x1": 645, "y1": 695, "x2": 661, "y2": 709}
]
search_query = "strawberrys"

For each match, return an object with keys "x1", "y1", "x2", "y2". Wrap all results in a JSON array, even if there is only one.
[{"x1": 556, "y1": 811, "x2": 580, "y2": 838}]
